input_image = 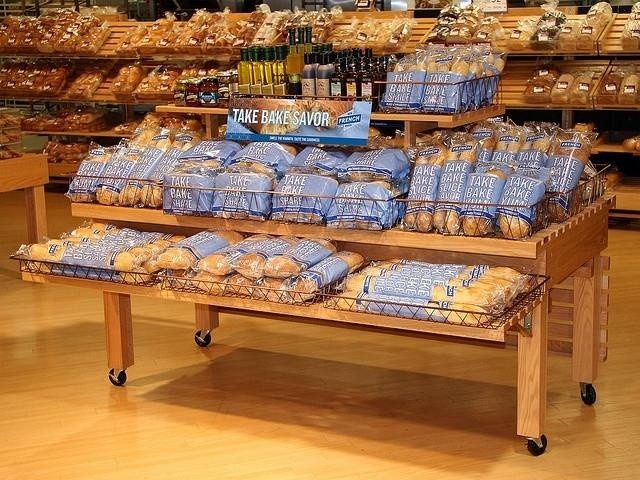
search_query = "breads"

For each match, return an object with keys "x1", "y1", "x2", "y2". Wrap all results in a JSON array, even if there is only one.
[
  {"x1": 0, "y1": 2, "x2": 640, "y2": 188},
  {"x1": 20, "y1": 45, "x2": 600, "y2": 325}
]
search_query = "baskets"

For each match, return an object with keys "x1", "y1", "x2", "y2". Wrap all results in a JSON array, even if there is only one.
[
  {"x1": 545, "y1": 164, "x2": 611, "y2": 223},
  {"x1": 161, "y1": 165, "x2": 614, "y2": 241},
  {"x1": 320, "y1": 271, "x2": 550, "y2": 331},
  {"x1": 160, "y1": 264, "x2": 366, "y2": 307},
  {"x1": 374, "y1": 74, "x2": 502, "y2": 115},
  {"x1": 395, "y1": 194, "x2": 555, "y2": 241},
  {"x1": 10, "y1": 253, "x2": 161, "y2": 286},
  {"x1": 71, "y1": 174, "x2": 161, "y2": 207},
  {"x1": 159, "y1": 184, "x2": 409, "y2": 232}
]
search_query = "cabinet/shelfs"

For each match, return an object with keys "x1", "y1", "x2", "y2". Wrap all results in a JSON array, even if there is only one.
[
  {"x1": 8, "y1": 156, "x2": 616, "y2": 455},
  {"x1": 0, "y1": 6, "x2": 640, "y2": 219}
]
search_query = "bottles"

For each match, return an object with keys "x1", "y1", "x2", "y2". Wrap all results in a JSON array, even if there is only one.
[{"x1": 173, "y1": 27, "x2": 389, "y2": 113}]
